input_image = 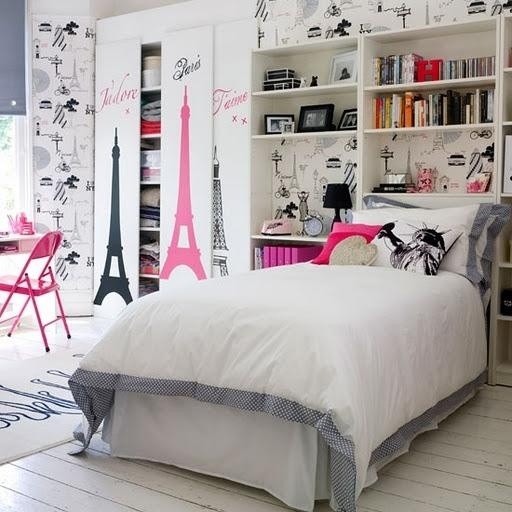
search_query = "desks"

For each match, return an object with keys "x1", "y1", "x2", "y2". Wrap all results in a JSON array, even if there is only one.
[{"x1": 1, "y1": 233, "x2": 57, "y2": 332}]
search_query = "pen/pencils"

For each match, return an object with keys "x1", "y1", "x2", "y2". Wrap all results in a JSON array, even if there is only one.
[{"x1": 6, "y1": 214, "x2": 18, "y2": 234}]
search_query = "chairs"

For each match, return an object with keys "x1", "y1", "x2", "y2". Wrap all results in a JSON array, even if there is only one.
[{"x1": 1, "y1": 229, "x2": 73, "y2": 352}]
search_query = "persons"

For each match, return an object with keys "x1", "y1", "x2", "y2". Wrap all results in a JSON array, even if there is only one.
[
  {"x1": 278, "y1": 119, "x2": 289, "y2": 131},
  {"x1": 339, "y1": 67, "x2": 350, "y2": 80},
  {"x1": 377, "y1": 227, "x2": 444, "y2": 276},
  {"x1": 271, "y1": 120, "x2": 278, "y2": 130}
]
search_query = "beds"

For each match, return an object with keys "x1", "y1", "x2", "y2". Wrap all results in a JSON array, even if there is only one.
[{"x1": 67, "y1": 194, "x2": 496, "y2": 510}]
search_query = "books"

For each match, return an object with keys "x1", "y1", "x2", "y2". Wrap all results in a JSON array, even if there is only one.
[
  {"x1": 369, "y1": 53, "x2": 497, "y2": 129},
  {"x1": 255, "y1": 245, "x2": 324, "y2": 271}
]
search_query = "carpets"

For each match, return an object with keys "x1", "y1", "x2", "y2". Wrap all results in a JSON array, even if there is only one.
[{"x1": 0, "y1": 341, "x2": 104, "y2": 464}]
